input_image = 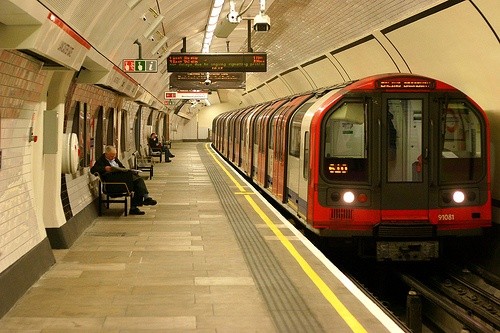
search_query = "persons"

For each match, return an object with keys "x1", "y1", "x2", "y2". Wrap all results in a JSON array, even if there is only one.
[
  {"x1": 90, "y1": 145, "x2": 157, "y2": 215},
  {"x1": 149, "y1": 133, "x2": 175, "y2": 162}
]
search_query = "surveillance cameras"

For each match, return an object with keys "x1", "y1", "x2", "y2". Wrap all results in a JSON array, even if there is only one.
[
  {"x1": 213, "y1": 13, "x2": 242, "y2": 38},
  {"x1": 191, "y1": 100, "x2": 197, "y2": 105},
  {"x1": 254, "y1": 14, "x2": 271, "y2": 32},
  {"x1": 205, "y1": 80, "x2": 210, "y2": 86}
]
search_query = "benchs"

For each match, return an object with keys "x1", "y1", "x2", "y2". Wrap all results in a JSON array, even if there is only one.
[
  {"x1": 147, "y1": 144, "x2": 162, "y2": 163},
  {"x1": 92, "y1": 172, "x2": 131, "y2": 216},
  {"x1": 132, "y1": 151, "x2": 155, "y2": 180},
  {"x1": 162, "y1": 136, "x2": 172, "y2": 148}
]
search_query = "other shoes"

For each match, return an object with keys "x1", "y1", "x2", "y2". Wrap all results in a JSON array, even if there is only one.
[
  {"x1": 169, "y1": 154, "x2": 175, "y2": 158},
  {"x1": 166, "y1": 158, "x2": 171, "y2": 162}
]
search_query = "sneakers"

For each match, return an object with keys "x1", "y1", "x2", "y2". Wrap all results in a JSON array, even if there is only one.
[
  {"x1": 143, "y1": 197, "x2": 157, "y2": 206},
  {"x1": 129, "y1": 208, "x2": 145, "y2": 215}
]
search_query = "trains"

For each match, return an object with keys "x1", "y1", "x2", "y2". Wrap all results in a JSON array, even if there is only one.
[{"x1": 210, "y1": 72, "x2": 492, "y2": 263}]
